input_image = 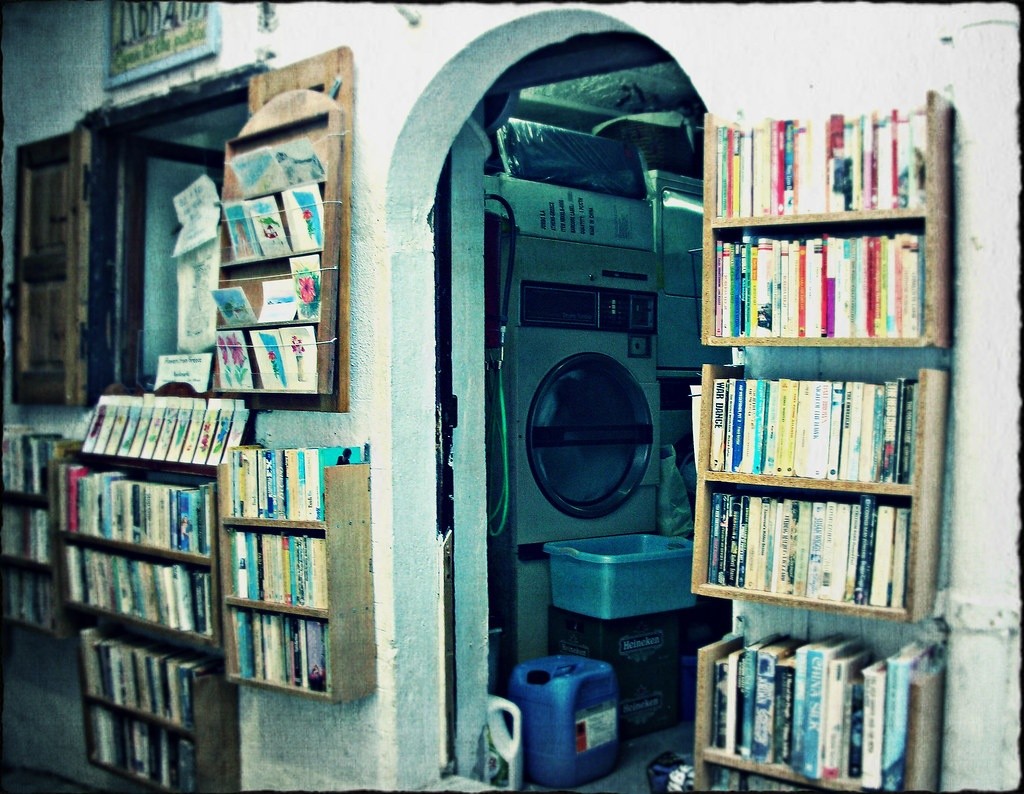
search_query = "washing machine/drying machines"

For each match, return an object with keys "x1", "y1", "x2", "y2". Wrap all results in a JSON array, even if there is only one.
[{"x1": 482, "y1": 234, "x2": 664, "y2": 544}]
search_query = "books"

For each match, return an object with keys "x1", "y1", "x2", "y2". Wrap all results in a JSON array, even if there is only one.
[
  {"x1": 715, "y1": 234, "x2": 925, "y2": 338},
  {"x1": 214, "y1": 137, "x2": 326, "y2": 392},
  {"x1": 711, "y1": 376, "x2": 917, "y2": 484},
  {"x1": 715, "y1": 108, "x2": 928, "y2": 217},
  {"x1": 222, "y1": 529, "x2": 332, "y2": 611},
  {"x1": 78, "y1": 627, "x2": 199, "y2": 794},
  {"x1": 221, "y1": 446, "x2": 362, "y2": 519},
  {"x1": 78, "y1": 393, "x2": 244, "y2": 468},
  {"x1": 0, "y1": 425, "x2": 63, "y2": 637},
  {"x1": 228, "y1": 605, "x2": 326, "y2": 694},
  {"x1": 707, "y1": 487, "x2": 911, "y2": 610},
  {"x1": 54, "y1": 468, "x2": 212, "y2": 641},
  {"x1": 711, "y1": 646, "x2": 928, "y2": 793}
]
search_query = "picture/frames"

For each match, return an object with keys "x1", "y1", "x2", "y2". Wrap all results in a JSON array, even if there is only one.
[{"x1": 101, "y1": 2, "x2": 221, "y2": 92}]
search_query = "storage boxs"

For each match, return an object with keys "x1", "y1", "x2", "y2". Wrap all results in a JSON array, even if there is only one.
[{"x1": 543, "y1": 534, "x2": 698, "y2": 620}]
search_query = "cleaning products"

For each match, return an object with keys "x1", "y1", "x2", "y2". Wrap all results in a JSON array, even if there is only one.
[{"x1": 484, "y1": 692, "x2": 522, "y2": 792}]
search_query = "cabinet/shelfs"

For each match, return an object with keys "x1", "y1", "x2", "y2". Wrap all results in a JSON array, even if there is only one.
[
  {"x1": 51, "y1": 446, "x2": 224, "y2": 650},
  {"x1": 689, "y1": 362, "x2": 952, "y2": 624},
  {"x1": 213, "y1": 43, "x2": 356, "y2": 415},
  {"x1": 0, "y1": 437, "x2": 85, "y2": 641},
  {"x1": 693, "y1": 635, "x2": 945, "y2": 792},
  {"x1": 217, "y1": 463, "x2": 377, "y2": 703},
  {"x1": 70, "y1": 621, "x2": 242, "y2": 794},
  {"x1": 700, "y1": 90, "x2": 957, "y2": 351}
]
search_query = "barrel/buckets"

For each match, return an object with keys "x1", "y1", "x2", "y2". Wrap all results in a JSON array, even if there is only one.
[{"x1": 507, "y1": 653, "x2": 621, "y2": 790}]
